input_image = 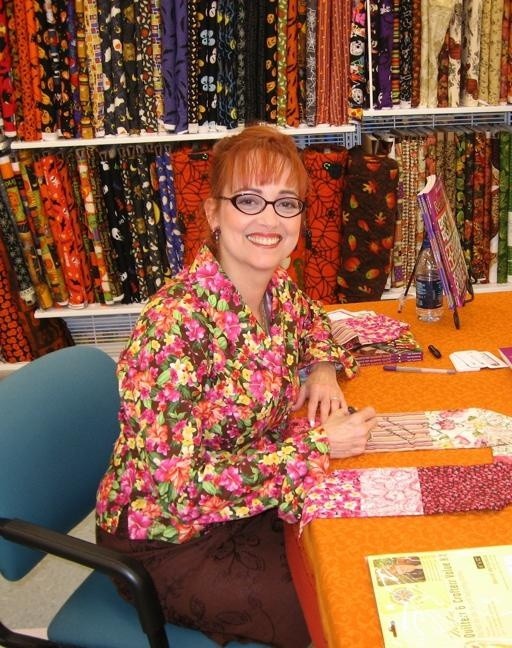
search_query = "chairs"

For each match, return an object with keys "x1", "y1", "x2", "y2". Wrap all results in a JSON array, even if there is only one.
[{"x1": 1, "y1": 345, "x2": 285, "y2": 647}]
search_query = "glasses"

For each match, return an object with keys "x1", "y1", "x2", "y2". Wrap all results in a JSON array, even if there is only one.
[{"x1": 213, "y1": 193, "x2": 310, "y2": 219}]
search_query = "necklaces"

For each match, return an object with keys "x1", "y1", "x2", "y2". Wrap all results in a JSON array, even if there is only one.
[{"x1": 249, "y1": 298, "x2": 266, "y2": 334}]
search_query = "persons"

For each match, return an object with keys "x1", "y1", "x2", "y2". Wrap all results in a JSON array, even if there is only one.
[{"x1": 93, "y1": 123, "x2": 378, "y2": 648}]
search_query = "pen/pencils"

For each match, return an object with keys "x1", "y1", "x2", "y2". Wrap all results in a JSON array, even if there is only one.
[
  {"x1": 382, "y1": 365, "x2": 457, "y2": 375},
  {"x1": 348, "y1": 406, "x2": 358, "y2": 413}
]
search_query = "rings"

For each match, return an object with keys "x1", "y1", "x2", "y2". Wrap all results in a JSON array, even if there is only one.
[
  {"x1": 329, "y1": 396, "x2": 341, "y2": 402},
  {"x1": 367, "y1": 430, "x2": 374, "y2": 442}
]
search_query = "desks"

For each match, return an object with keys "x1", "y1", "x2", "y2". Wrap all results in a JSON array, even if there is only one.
[{"x1": 273, "y1": 288, "x2": 512, "y2": 647}]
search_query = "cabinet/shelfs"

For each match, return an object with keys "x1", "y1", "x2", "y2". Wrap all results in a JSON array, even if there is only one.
[
  {"x1": 7, "y1": 121, "x2": 360, "y2": 364},
  {"x1": 345, "y1": 100, "x2": 512, "y2": 302}
]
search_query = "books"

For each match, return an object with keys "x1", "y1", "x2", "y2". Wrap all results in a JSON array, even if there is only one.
[{"x1": 416, "y1": 174, "x2": 469, "y2": 310}]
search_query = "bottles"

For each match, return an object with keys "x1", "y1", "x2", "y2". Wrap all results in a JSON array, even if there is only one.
[{"x1": 416, "y1": 241, "x2": 443, "y2": 321}]
